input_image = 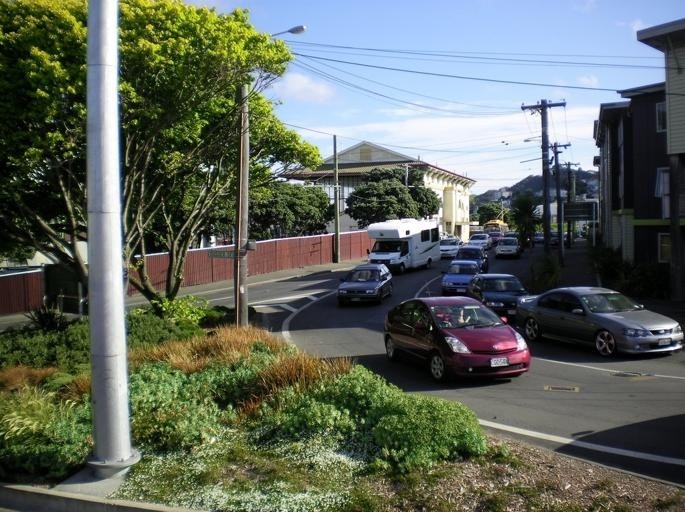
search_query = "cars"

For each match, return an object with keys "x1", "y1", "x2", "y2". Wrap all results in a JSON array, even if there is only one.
[
  {"x1": 440, "y1": 261, "x2": 483, "y2": 296},
  {"x1": 338, "y1": 264, "x2": 396, "y2": 305},
  {"x1": 467, "y1": 273, "x2": 532, "y2": 320},
  {"x1": 383, "y1": 296, "x2": 533, "y2": 380},
  {"x1": 518, "y1": 286, "x2": 684, "y2": 359},
  {"x1": 440, "y1": 231, "x2": 571, "y2": 260},
  {"x1": 456, "y1": 246, "x2": 490, "y2": 272}
]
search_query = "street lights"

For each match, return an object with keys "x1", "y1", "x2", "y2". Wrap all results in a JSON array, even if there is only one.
[{"x1": 236, "y1": 22, "x2": 310, "y2": 331}]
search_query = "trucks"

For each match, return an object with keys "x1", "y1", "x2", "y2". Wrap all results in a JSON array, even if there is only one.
[{"x1": 366, "y1": 218, "x2": 442, "y2": 277}]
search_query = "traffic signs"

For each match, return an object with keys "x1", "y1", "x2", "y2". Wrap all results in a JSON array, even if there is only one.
[{"x1": 206, "y1": 250, "x2": 238, "y2": 259}]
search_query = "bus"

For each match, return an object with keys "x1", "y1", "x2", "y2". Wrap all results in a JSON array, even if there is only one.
[{"x1": 484, "y1": 219, "x2": 510, "y2": 231}]
